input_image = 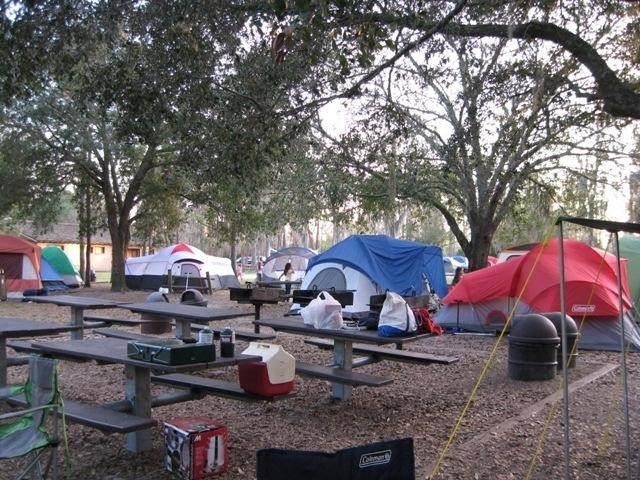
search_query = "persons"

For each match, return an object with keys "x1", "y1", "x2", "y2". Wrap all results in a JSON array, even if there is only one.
[
  {"x1": 235, "y1": 263, "x2": 243, "y2": 281},
  {"x1": 283, "y1": 262, "x2": 295, "y2": 302},
  {"x1": 256, "y1": 260, "x2": 264, "y2": 288}
]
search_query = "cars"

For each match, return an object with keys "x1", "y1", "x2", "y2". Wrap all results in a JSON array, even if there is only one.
[{"x1": 236, "y1": 256, "x2": 252, "y2": 264}]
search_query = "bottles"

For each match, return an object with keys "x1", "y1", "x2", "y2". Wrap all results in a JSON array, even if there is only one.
[{"x1": 198, "y1": 325, "x2": 236, "y2": 357}]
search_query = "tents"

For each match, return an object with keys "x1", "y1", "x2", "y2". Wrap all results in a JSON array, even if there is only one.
[
  {"x1": 39, "y1": 245, "x2": 86, "y2": 290},
  {"x1": 123, "y1": 242, "x2": 240, "y2": 293},
  {"x1": 433, "y1": 237, "x2": 640, "y2": 352},
  {"x1": 283, "y1": 234, "x2": 449, "y2": 327},
  {"x1": 0, "y1": 234, "x2": 44, "y2": 294},
  {"x1": 612, "y1": 236, "x2": 640, "y2": 326},
  {"x1": 252, "y1": 247, "x2": 318, "y2": 288},
  {"x1": 442, "y1": 242, "x2": 539, "y2": 288}
]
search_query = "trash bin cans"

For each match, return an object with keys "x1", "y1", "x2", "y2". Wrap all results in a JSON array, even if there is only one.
[
  {"x1": 0, "y1": 267, "x2": 8, "y2": 301},
  {"x1": 507, "y1": 312, "x2": 581, "y2": 382},
  {"x1": 140, "y1": 291, "x2": 172, "y2": 335},
  {"x1": 180, "y1": 288, "x2": 209, "y2": 333}
]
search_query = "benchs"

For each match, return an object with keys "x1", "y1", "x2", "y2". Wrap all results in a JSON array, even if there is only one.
[
  {"x1": 6, "y1": 315, "x2": 461, "y2": 439},
  {"x1": 284, "y1": 293, "x2": 293, "y2": 298}
]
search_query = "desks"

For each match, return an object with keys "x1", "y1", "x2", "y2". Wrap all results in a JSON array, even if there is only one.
[
  {"x1": 261, "y1": 280, "x2": 302, "y2": 294},
  {"x1": 0, "y1": 317, "x2": 82, "y2": 387},
  {"x1": 25, "y1": 295, "x2": 134, "y2": 340},
  {"x1": 121, "y1": 299, "x2": 259, "y2": 338}
]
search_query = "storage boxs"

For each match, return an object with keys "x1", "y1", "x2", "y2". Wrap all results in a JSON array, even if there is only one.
[{"x1": 163, "y1": 415, "x2": 230, "y2": 479}]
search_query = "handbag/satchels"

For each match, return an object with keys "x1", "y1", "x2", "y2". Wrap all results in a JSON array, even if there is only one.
[{"x1": 376, "y1": 285, "x2": 420, "y2": 337}]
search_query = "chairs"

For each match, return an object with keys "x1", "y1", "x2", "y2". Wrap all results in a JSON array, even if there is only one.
[{"x1": 0, "y1": 357, "x2": 60, "y2": 480}]
